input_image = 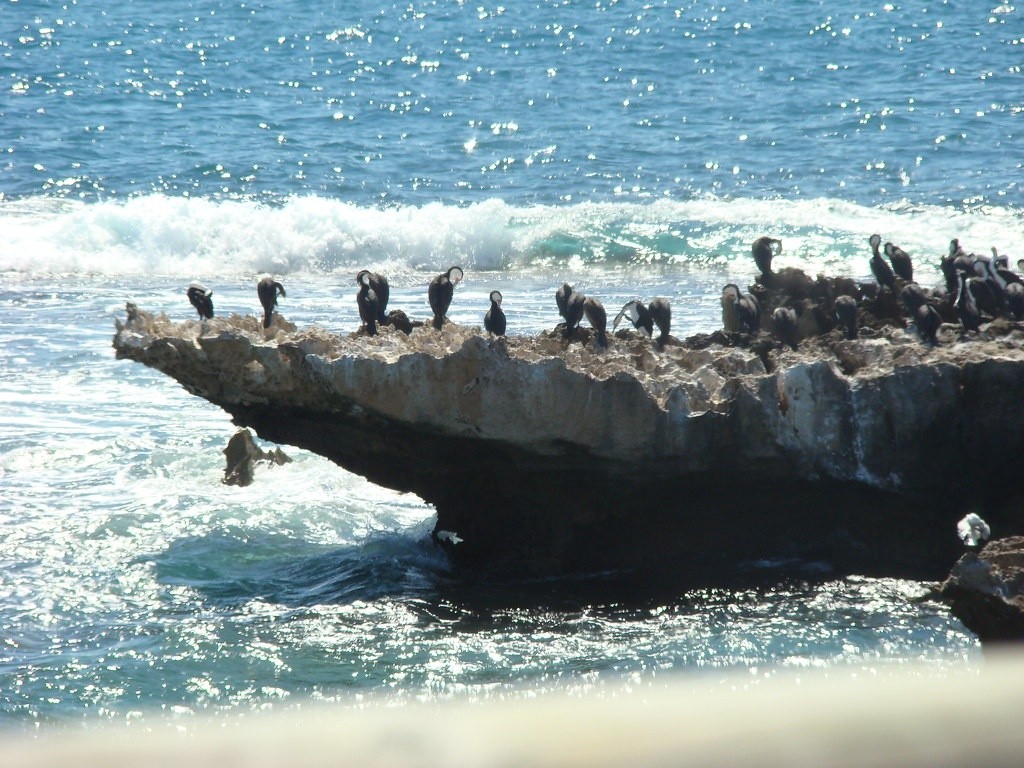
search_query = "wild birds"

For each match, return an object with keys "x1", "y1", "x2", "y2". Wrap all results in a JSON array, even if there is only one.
[
  {"x1": 355, "y1": 270, "x2": 389, "y2": 336},
  {"x1": 555, "y1": 233, "x2": 1024, "y2": 343},
  {"x1": 484, "y1": 290, "x2": 507, "y2": 337},
  {"x1": 258, "y1": 277, "x2": 286, "y2": 329},
  {"x1": 427, "y1": 266, "x2": 463, "y2": 322},
  {"x1": 955, "y1": 512, "x2": 991, "y2": 546},
  {"x1": 186, "y1": 280, "x2": 213, "y2": 320}
]
